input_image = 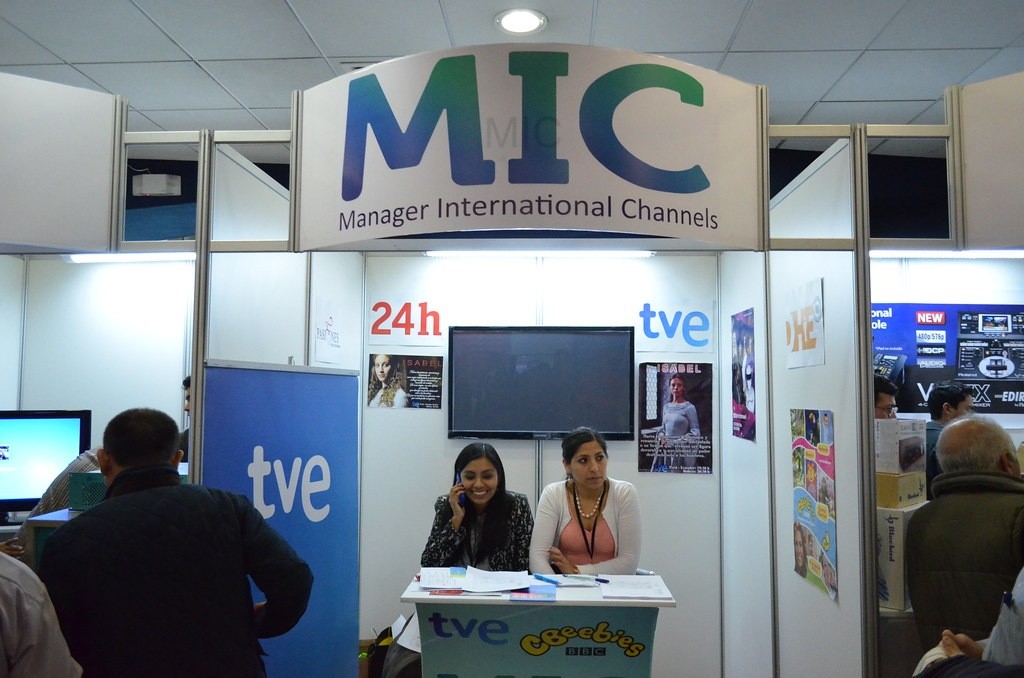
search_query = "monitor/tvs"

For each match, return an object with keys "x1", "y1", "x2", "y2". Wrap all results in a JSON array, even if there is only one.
[
  {"x1": 978, "y1": 314, "x2": 1012, "y2": 333},
  {"x1": 0, "y1": 409, "x2": 92, "y2": 526},
  {"x1": 448, "y1": 325, "x2": 635, "y2": 441}
]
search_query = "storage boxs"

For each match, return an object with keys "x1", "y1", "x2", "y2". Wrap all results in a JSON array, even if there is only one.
[
  {"x1": 69, "y1": 472, "x2": 187, "y2": 512},
  {"x1": 873, "y1": 419, "x2": 931, "y2": 611}
]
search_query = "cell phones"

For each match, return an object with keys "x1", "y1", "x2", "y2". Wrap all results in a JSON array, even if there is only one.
[{"x1": 456, "y1": 473, "x2": 464, "y2": 507}]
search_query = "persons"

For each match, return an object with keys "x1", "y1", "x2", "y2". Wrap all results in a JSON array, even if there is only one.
[
  {"x1": 789, "y1": 410, "x2": 837, "y2": 595},
  {"x1": 732, "y1": 331, "x2": 755, "y2": 441},
  {"x1": 1, "y1": 369, "x2": 313, "y2": 678},
  {"x1": 368, "y1": 355, "x2": 407, "y2": 408},
  {"x1": 528, "y1": 428, "x2": 642, "y2": 576},
  {"x1": 657, "y1": 373, "x2": 700, "y2": 473},
  {"x1": 420, "y1": 443, "x2": 533, "y2": 575},
  {"x1": 873, "y1": 374, "x2": 1024, "y2": 677}
]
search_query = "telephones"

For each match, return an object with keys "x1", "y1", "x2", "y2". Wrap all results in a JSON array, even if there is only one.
[{"x1": 873, "y1": 353, "x2": 908, "y2": 382}]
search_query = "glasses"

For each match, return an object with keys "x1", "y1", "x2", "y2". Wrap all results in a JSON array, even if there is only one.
[{"x1": 877, "y1": 406, "x2": 898, "y2": 417}]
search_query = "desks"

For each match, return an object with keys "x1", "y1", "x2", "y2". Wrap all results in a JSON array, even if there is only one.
[
  {"x1": 401, "y1": 571, "x2": 677, "y2": 678},
  {"x1": 877, "y1": 607, "x2": 924, "y2": 678},
  {"x1": 26, "y1": 509, "x2": 86, "y2": 576}
]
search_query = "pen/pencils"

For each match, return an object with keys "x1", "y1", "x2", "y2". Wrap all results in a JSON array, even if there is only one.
[
  {"x1": 1001, "y1": 590, "x2": 1012, "y2": 610},
  {"x1": 563, "y1": 573, "x2": 609, "y2": 583},
  {"x1": 532, "y1": 573, "x2": 561, "y2": 584}
]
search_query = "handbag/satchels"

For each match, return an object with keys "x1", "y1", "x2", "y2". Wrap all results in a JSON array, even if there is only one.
[
  {"x1": 649, "y1": 446, "x2": 674, "y2": 472},
  {"x1": 367, "y1": 627, "x2": 393, "y2": 678},
  {"x1": 358, "y1": 655, "x2": 368, "y2": 678}
]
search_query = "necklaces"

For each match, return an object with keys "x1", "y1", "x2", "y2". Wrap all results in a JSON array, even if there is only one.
[{"x1": 575, "y1": 483, "x2": 604, "y2": 518}]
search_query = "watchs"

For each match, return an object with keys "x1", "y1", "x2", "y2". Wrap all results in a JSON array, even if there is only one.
[{"x1": 573, "y1": 567, "x2": 578, "y2": 574}]
